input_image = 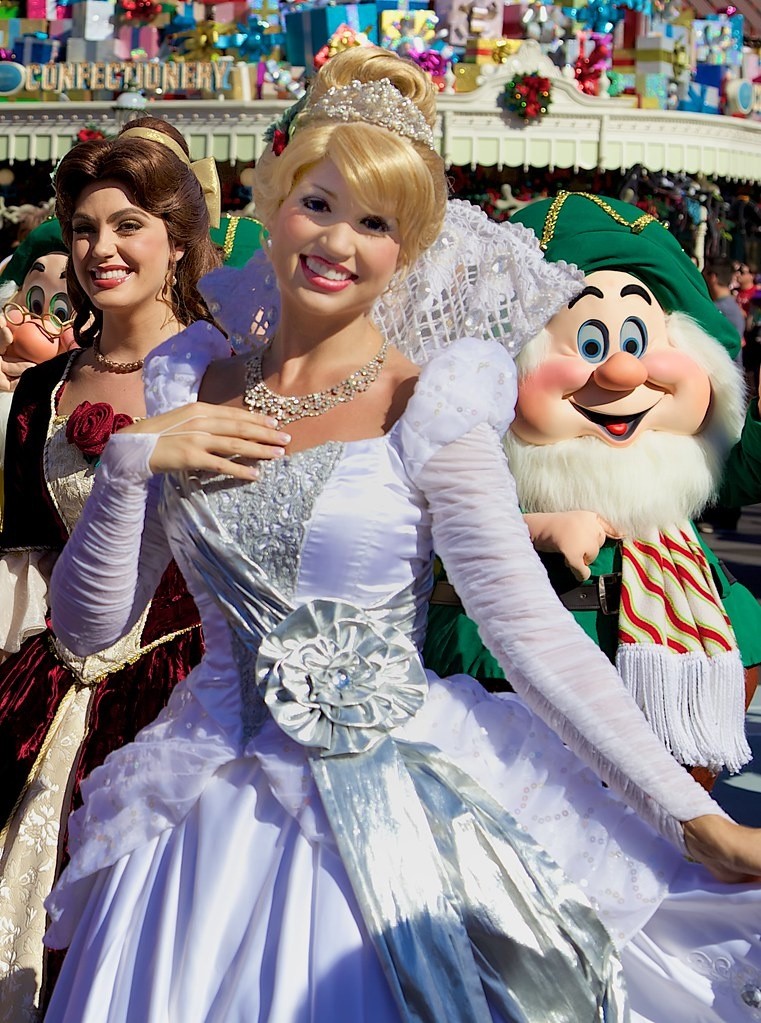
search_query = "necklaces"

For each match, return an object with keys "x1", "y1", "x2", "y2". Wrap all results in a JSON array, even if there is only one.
[
  {"x1": 244, "y1": 334, "x2": 390, "y2": 432},
  {"x1": 93, "y1": 334, "x2": 144, "y2": 373}
]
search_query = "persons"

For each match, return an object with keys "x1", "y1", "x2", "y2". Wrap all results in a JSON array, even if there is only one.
[
  {"x1": 1, "y1": 218, "x2": 99, "y2": 457},
  {"x1": 419, "y1": 190, "x2": 760, "y2": 796},
  {"x1": 38, "y1": 45, "x2": 761, "y2": 1023},
  {"x1": 0, "y1": 116, "x2": 238, "y2": 1023},
  {"x1": 702, "y1": 255, "x2": 761, "y2": 403}
]
